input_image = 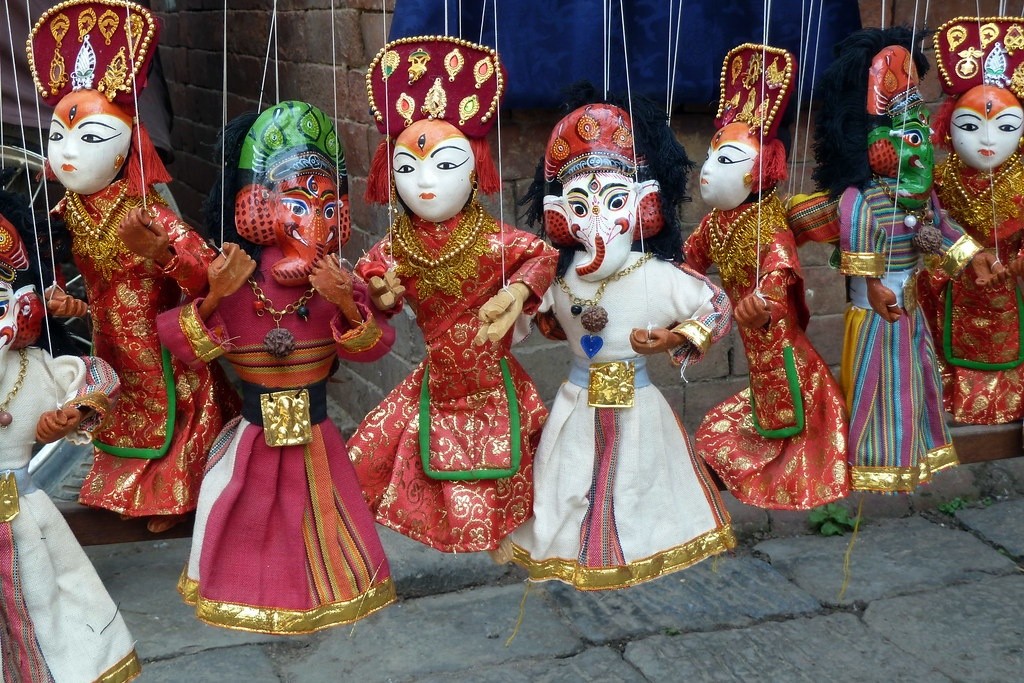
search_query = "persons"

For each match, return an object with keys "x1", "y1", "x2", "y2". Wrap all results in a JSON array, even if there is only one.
[
  {"x1": 920, "y1": 15, "x2": 1024, "y2": 427},
  {"x1": 509, "y1": 78, "x2": 737, "y2": 592},
  {"x1": 0, "y1": 0, "x2": 244, "y2": 536},
  {"x1": 680, "y1": 42, "x2": 850, "y2": 511}
]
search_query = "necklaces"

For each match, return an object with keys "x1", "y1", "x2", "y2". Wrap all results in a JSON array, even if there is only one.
[
  {"x1": 553, "y1": 252, "x2": 654, "y2": 359},
  {"x1": 872, "y1": 172, "x2": 943, "y2": 254},
  {"x1": 946, "y1": 152, "x2": 1022, "y2": 215},
  {"x1": 391, "y1": 203, "x2": 485, "y2": 267},
  {"x1": 708, "y1": 186, "x2": 777, "y2": 254},
  {"x1": 0, "y1": 348, "x2": 29, "y2": 428},
  {"x1": 246, "y1": 278, "x2": 316, "y2": 362},
  {"x1": 65, "y1": 176, "x2": 130, "y2": 240}
]
